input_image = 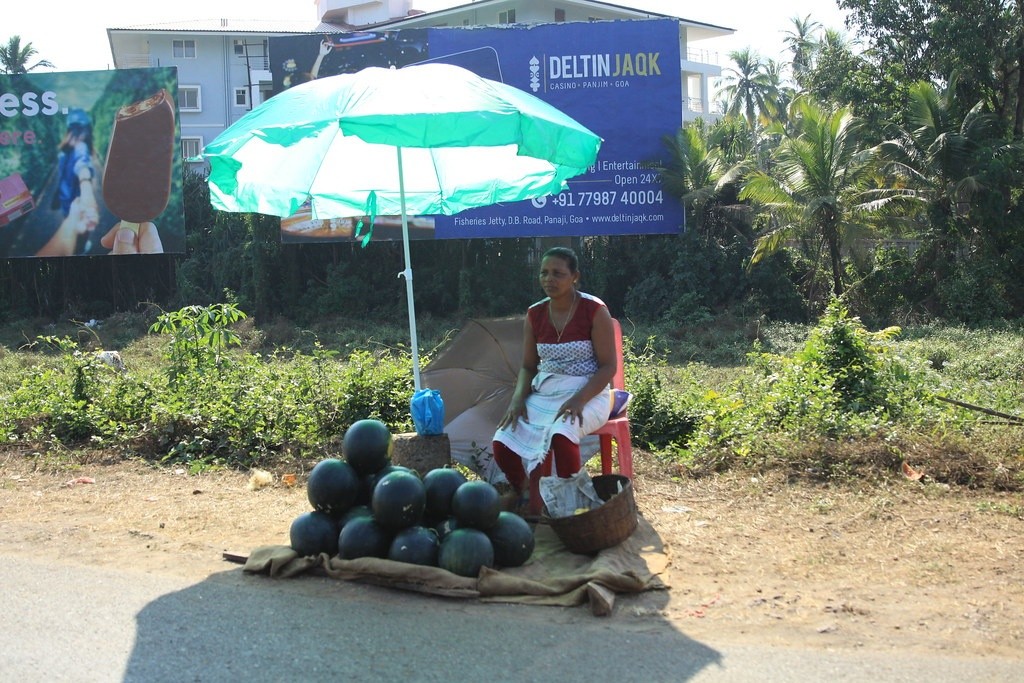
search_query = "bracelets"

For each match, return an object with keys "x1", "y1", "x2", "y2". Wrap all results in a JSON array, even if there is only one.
[{"x1": 78, "y1": 178, "x2": 92, "y2": 183}]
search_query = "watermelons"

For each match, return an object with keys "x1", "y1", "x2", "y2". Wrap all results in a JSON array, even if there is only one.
[{"x1": 290, "y1": 420, "x2": 535, "y2": 578}]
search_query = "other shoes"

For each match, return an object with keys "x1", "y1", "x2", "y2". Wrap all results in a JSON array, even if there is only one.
[{"x1": 525, "y1": 495, "x2": 545, "y2": 523}]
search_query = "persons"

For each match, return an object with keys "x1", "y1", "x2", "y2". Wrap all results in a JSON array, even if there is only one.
[
  {"x1": 31, "y1": 109, "x2": 163, "y2": 256},
  {"x1": 493, "y1": 247, "x2": 616, "y2": 517},
  {"x1": 290, "y1": 39, "x2": 332, "y2": 86}
]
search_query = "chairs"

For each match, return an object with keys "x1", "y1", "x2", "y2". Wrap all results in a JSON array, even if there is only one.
[{"x1": 530, "y1": 318, "x2": 633, "y2": 518}]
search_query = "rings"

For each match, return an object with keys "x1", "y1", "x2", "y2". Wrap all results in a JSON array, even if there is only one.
[{"x1": 565, "y1": 408, "x2": 572, "y2": 414}]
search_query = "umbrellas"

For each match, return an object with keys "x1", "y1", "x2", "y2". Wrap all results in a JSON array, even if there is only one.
[
  {"x1": 201, "y1": 63, "x2": 604, "y2": 394},
  {"x1": 419, "y1": 315, "x2": 603, "y2": 499}
]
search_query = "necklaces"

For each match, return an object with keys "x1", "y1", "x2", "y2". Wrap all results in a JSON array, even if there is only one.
[{"x1": 546, "y1": 293, "x2": 575, "y2": 344}]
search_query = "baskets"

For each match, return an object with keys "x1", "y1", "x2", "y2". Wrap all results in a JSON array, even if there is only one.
[{"x1": 540, "y1": 475, "x2": 636, "y2": 554}]
